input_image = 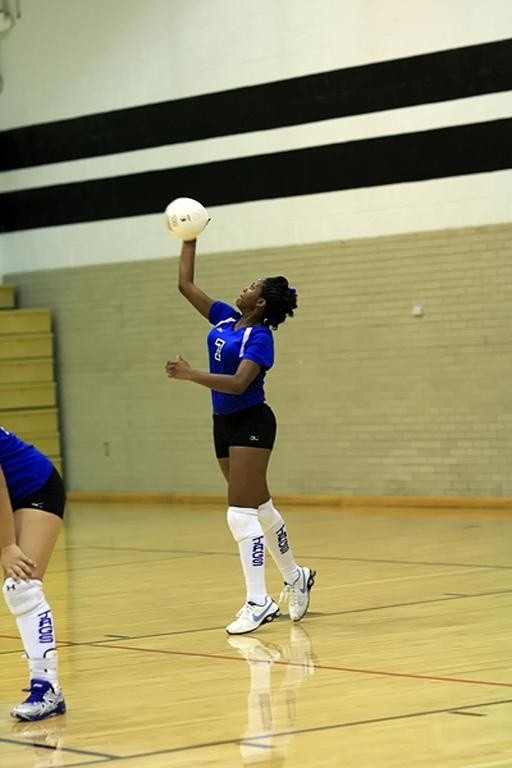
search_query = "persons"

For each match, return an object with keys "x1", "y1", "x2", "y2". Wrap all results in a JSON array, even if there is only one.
[
  {"x1": 228, "y1": 624, "x2": 316, "y2": 767},
  {"x1": 165, "y1": 217, "x2": 316, "y2": 634},
  {"x1": 1, "y1": 425, "x2": 67, "y2": 722},
  {"x1": 12, "y1": 716, "x2": 66, "y2": 768}
]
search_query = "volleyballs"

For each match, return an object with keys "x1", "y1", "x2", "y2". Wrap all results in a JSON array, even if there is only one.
[{"x1": 163, "y1": 197, "x2": 211, "y2": 242}]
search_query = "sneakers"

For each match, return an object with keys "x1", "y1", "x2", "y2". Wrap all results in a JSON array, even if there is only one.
[
  {"x1": 283, "y1": 564, "x2": 317, "y2": 622},
  {"x1": 225, "y1": 593, "x2": 281, "y2": 636},
  {"x1": 10, "y1": 678, "x2": 68, "y2": 722}
]
